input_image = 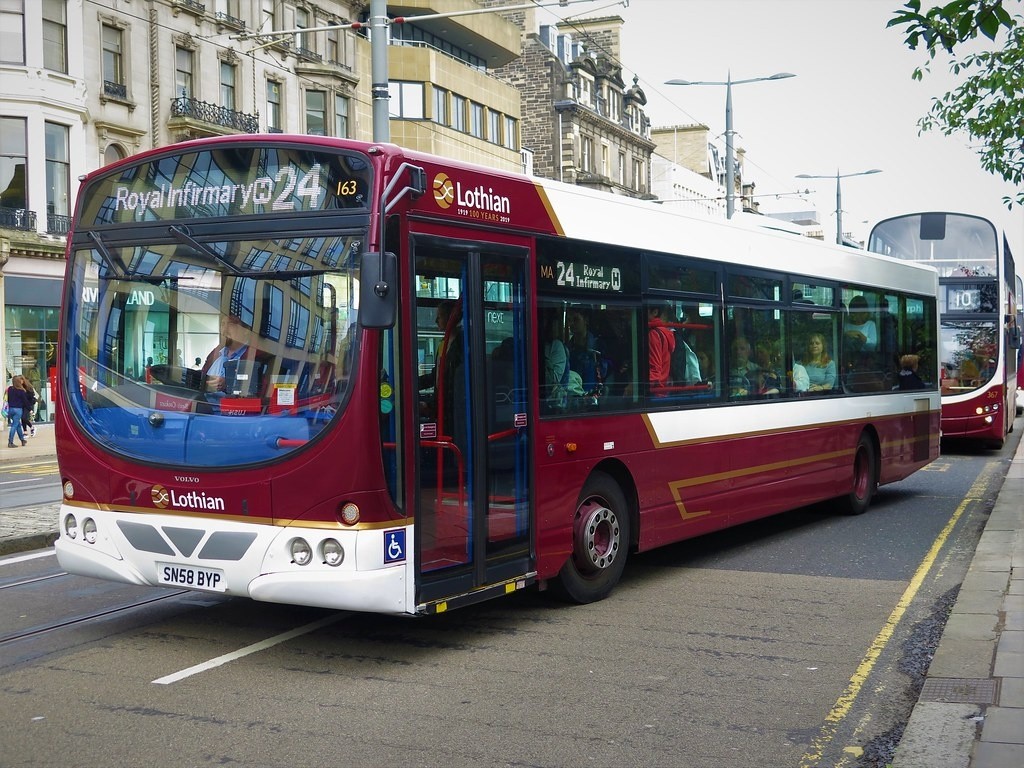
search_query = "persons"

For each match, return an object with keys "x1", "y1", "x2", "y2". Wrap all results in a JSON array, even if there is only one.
[
  {"x1": 415, "y1": 300, "x2": 466, "y2": 479},
  {"x1": 2, "y1": 375, "x2": 26, "y2": 450},
  {"x1": 969, "y1": 326, "x2": 999, "y2": 386},
  {"x1": 192, "y1": 356, "x2": 203, "y2": 374},
  {"x1": 844, "y1": 295, "x2": 911, "y2": 390},
  {"x1": 333, "y1": 317, "x2": 370, "y2": 395},
  {"x1": 724, "y1": 279, "x2": 787, "y2": 398},
  {"x1": 14, "y1": 374, "x2": 38, "y2": 440},
  {"x1": 646, "y1": 270, "x2": 716, "y2": 396},
  {"x1": 123, "y1": 366, "x2": 137, "y2": 383},
  {"x1": 177, "y1": 348, "x2": 186, "y2": 369},
  {"x1": 535, "y1": 306, "x2": 633, "y2": 409},
  {"x1": 490, "y1": 338, "x2": 516, "y2": 363},
  {"x1": 794, "y1": 287, "x2": 836, "y2": 395},
  {"x1": 145, "y1": 355, "x2": 155, "y2": 375},
  {"x1": 200, "y1": 311, "x2": 267, "y2": 402}
]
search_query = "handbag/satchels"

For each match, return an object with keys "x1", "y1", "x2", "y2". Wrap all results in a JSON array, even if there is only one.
[
  {"x1": 2, "y1": 388, "x2": 9, "y2": 417},
  {"x1": 41, "y1": 396, "x2": 46, "y2": 410}
]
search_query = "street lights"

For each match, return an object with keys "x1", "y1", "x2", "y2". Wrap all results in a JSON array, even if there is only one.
[
  {"x1": 664, "y1": 66, "x2": 795, "y2": 218},
  {"x1": 795, "y1": 166, "x2": 885, "y2": 308}
]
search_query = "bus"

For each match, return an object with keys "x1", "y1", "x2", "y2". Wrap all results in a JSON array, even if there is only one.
[
  {"x1": 866, "y1": 208, "x2": 1024, "y2": 451},
  {"x1": 53, "y1": 132, "x2": 943, "y2": 619}
]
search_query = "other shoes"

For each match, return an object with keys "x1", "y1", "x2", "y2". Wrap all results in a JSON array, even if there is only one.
[
  {"x1": 22, "y1": 440, "x2": 27, "y2": 445},
  {"x1": 31, "y1": 428, "x2": 35, "y2": 437},
  {"x1": 23, "y1": 434, "x2": 30, "y2": 439},
  {"x1": 8, "y1": 444, "x2": 18, "y2": 448}
]
